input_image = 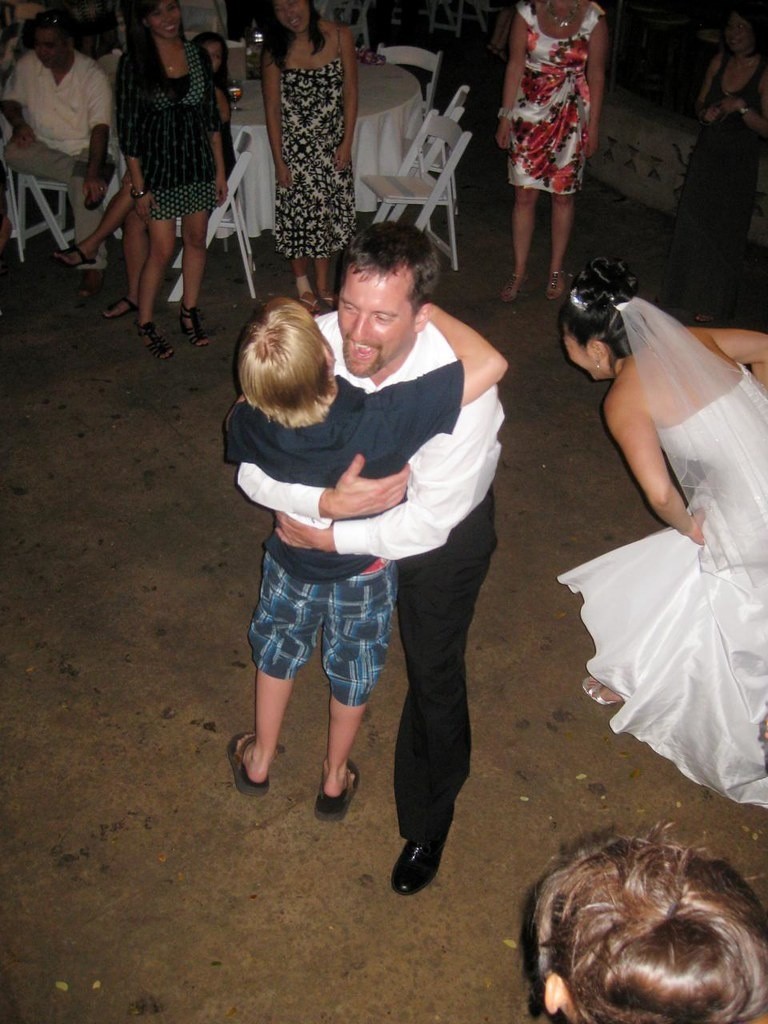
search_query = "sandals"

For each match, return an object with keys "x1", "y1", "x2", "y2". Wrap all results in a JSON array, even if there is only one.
[
  {"x1": 582, "y1": 676, "x2": 623, "y2": 705},
  {"x1": 136, "y1": 318, "x2": 174, "y2": 359},
  {"x1": 179, "y1": 300, "x2": 209, "y2": 347}
]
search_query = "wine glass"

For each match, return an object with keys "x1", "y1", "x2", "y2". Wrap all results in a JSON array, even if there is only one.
[{"x1": 226, "y1": 79, "x2": 243, "y2": 111}]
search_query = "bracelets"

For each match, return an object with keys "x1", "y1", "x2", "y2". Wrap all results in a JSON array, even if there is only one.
[
  {"x1": 130, "y1": 185, "x2": 150, "y2": 199},
  {"x1": 497, "y1": 108, "x2": 511, "y2": 121},
  {"x1": 739, "y1": 105, "x2": 751, "y2": 116},
  {"x1": 699, "y1": 109, "x2": 712, "y2": 126}
]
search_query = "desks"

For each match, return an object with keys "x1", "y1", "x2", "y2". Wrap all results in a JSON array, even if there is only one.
[{"x1": 230, "y1": 64, "x2": 428, "y2": 238}]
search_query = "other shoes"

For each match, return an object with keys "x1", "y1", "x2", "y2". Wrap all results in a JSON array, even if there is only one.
[
  {"x1": 78, "y1": 270, "x2": 104, "y2": 299},
  {"x1": 87, "y1": 159, "x2": 116, "y2": 210}
]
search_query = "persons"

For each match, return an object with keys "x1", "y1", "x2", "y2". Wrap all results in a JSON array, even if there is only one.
[
  {"x1": 114, "y1": 0, "x2": 229, "y2": 360},
  {"x1": 487, "y1": 7, "x2": 516, "y2": 54},
  {"x1": 371, "y1": 0, "x2": 421, "y2": 45},
  {"x1": 496, "y1": 0, "x2": 610, "y2": 303},
  {"x1": 0, "y1": 0, "x2": 119, "y2": 305},
  {"x1": 519, "y1": 819, "x2": 767, "y2": 1023},
  {"x1": 557, "y1": 254, "x2": 768, "y2": 810},
  {"x1": 226, "y1": 298, "x2": 509, "y2": 820},
  {"x1": 657, "y1": 3, "x2": 768, "y2": 322},
  {"x1": 51, "y1": 30, "x2": 238, "y2": 318},
  {"x1": 253, "y1": 0, "x2": 359, "y2": 315},
  {"x1": 236, "y1": 217, "x2": 506, "y2": 896}
]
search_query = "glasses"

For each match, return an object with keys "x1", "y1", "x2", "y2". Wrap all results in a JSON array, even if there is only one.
[{"x1": 35, "y1": 14, "x2": 61, "y2": 27}]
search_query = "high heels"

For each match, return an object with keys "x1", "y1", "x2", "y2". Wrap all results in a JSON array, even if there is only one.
[
  {"x1": 546, "y1": 269, "x2": 564, "y2": 301},
  {"x1": 501, "y1": 272, "x2": 527, "y2": 304}
]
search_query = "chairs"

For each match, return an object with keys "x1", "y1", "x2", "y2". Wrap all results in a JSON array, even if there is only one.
[{"x1": 0, "y1": 36, "x2": 473, "y2": 303}]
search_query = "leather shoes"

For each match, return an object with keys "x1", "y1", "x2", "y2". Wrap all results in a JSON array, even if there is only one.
[{"x1": 393, "y1": 811, "x2": 445, "y2": 894}]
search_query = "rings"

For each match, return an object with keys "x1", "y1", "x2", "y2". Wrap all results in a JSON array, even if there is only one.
[{"x1": 99, "y1": 187, "x2": 103, "y2": 191}]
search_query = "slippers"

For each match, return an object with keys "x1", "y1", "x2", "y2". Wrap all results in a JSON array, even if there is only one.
[
  {"x1": 315, "y1": 757, "x2": 358, "y2": 824},
  {"x1": 226, "y1": 729, "x2": 272, "y2": 796},
  {"x1": 101, "y1": 296, "x2": 138, "y2": 317},
  {"x1": 321, "y1": 291, "x2": 339, "y2": 310},
  {"x1": 55, "y1": 242, "x2": 97, "y2": 266},
  {"x1": 298, "y1": 288, "x2": 321, "y2": 316}
]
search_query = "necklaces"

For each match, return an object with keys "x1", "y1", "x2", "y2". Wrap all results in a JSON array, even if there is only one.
[
  {"x1": 735, "y1": 56, "x2": 756, "y2": 70},
  {"x1": 160, "y1": 41, "x2": 178, "y2": 71},
  {"x1": 545, "y1": 0, "x2": 579, "y2": 27}
]
